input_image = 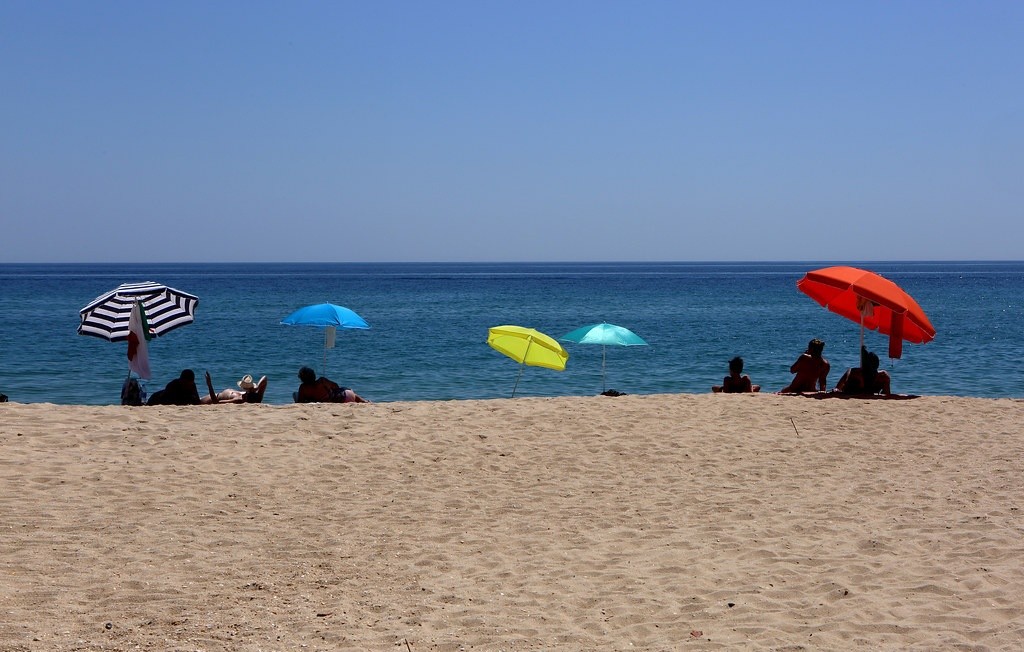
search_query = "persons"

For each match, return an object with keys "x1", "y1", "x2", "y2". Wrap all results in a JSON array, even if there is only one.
[
  {"x1": 779, "y1": 339, "x2": 830, "y2": 393},
  {"x1": 145, "y1": 369, "x2": 201, "y2": 406},
  {"x1": 711, "y1": 357, "x2": 761, "y2": 393},
  {"x1": 836, "y1": 352, "x2": 891, "y2": 395},
  {"x1": 292, "y1": 367, "x2": 370, "y2": 403},
  {"x1": 199, "y1": 373, "x2": 267, "y2": 405}
]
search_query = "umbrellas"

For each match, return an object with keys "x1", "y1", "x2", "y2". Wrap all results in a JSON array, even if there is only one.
[
  {"x1": 486, "y1": 325, "x2": 570, "y2": 399},
  {"x1": 75, "y1": 280, "x2": 200, "y2": 385},
  {"x1": 796, "y1": 266, "x2": 936, "y2": 369},
  {"x1": 277, "y1": 302, "x2": 372, "y2": 378},
  {"x1": 557, "y1": 320, "x2": 649, "y2": 393}
]
